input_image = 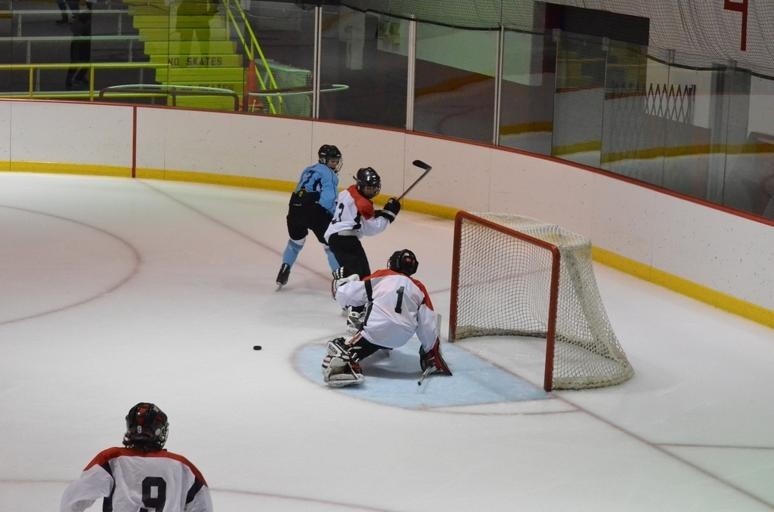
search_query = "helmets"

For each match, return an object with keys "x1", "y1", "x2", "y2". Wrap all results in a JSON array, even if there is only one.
[
  {"x1": 124, "y1": 402, "x2": 169, "y2": 453},
  {"x1": 318, "y1": 143, "x2": 344, "y2": 174},
  {"x1": 387, "y1": 249, "x2": 419, "y2": 278},
  {"x1": 356, "y1": 167, "x2": 382, "y2": 200}
]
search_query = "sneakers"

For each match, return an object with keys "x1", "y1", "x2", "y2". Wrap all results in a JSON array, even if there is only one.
[
  {"x1": 346, "y1": 311, "x2": 364, "y2": 333},
  {"x1": 328, "y1": 369, "x2": 364, "y2": 386}
]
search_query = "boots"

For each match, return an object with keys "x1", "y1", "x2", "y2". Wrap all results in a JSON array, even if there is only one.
[{"x1": 275, "y1": 263, "x2": 291, "y2": 286}]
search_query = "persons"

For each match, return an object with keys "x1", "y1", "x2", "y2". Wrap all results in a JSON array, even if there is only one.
[
  {"x1": 59, "y1": 402, "x2": 215, "y2": 512},
  {"x1": 324, "y1": 166, "x2": 400, "y2": 333},
  {"x1": 275, "y1": 145, "x2": 345, "y2": 298},
  {"x1": 323, "y1": 248, "x2": 452, "y2": 388}
]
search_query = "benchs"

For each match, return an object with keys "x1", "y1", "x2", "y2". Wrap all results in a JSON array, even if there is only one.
[{"x1": 0, "y1": 0, "x2": 167, "y2": 107}]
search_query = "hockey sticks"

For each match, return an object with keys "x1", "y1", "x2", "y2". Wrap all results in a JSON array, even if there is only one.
[
  {"x1": 396, "y1": 160, "x2": 432, "y2": 201},
  {"x1": 418, "y1": 314, "x2": 441, "y2": 384}
]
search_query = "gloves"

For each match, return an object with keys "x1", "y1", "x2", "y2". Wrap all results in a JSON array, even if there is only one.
[
  {"x1": 331, "y1": 265, "x2": 349, "y2": 284},
  {"x1": 380, "y1": 196, "x2": 401, "y2": 224}
]
